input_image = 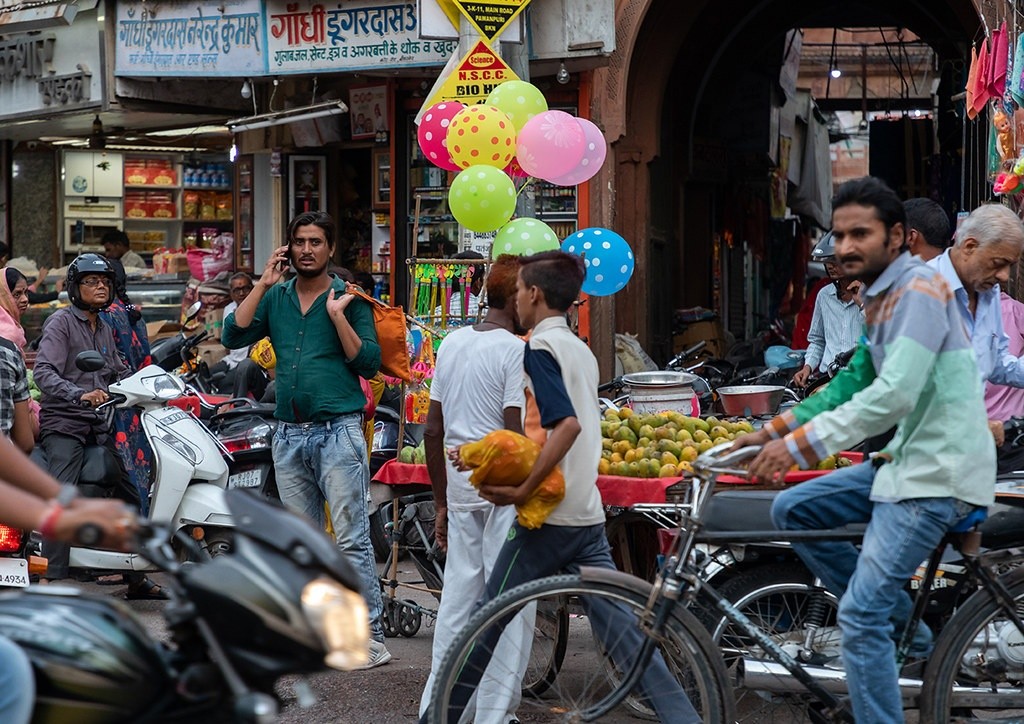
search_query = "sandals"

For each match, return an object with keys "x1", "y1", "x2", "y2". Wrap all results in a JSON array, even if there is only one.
[{"x1": 122, "y1": 576, "x2": 172, "y2": 601}]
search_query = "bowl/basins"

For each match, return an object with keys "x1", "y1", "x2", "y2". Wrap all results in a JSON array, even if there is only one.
[
  {"x1": 716, "y1": 383, "x2": 786, "y2": 415},
  {"x1": 623, "y1": 371, "x2": 699, "y2": 388}
]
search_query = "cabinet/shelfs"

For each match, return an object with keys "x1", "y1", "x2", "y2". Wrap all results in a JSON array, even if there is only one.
[
  {"x1": 65, "y1": 151, "x2": 123, "y2": 199},
  {"x1": 122, "y1": 149, "x2": 236, "y2": 270},
  {"x1": 21, "y1": 269, "x2": 190, "y2": 355}
]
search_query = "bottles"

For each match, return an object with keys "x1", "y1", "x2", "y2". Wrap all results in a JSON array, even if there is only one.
[{"x1": 183, "y1": 166, "x2": 230, "y2": 188}]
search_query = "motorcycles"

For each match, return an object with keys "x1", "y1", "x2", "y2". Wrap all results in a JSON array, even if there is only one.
[{"x1": 628, "y1": 414, "x2": 1024, "y2": 724}]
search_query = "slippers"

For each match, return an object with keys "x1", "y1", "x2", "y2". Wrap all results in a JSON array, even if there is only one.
[{"x1": 96, "y1": 576, "x2": 123, "y2": 585}]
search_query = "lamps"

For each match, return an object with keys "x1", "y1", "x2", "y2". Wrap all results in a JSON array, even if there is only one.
[{"x1": 226, "y1": 99, "x2": 349, "y2": 133}]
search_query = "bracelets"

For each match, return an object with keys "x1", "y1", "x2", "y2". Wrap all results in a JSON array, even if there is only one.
[
  {"x1": 36, "y1": 499, "x2": 63, "y2": 538},
  {"x1": 56, "y1": 482, "x2": 81, "y2": 508}
]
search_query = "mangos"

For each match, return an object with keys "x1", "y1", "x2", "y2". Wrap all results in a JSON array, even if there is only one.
[
  {"x1": 400, "y1": 440, "x2": 427, "y2": 465},
  {"x1": 600, "y1": 409, "x2": 850, "y2": 479}
]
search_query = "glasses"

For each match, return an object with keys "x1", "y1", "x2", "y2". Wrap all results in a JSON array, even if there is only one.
[
  {"x1": 79, "y1": 278, "x2": 112, "y2": 288},
  {"x1": 232, "y1": 285, "x2": 252, "y2": 293}
]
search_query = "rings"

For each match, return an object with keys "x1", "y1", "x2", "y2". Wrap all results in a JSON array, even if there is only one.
[
  {"x1": 117, "y1": 518, "x2": 130, "y2": 533},
  {"x1": 125, "y1": 504, "x2": 137, "y2": 520}
]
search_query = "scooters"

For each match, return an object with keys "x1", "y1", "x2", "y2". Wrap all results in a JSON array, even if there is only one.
[{"x1": 2, "y1": 301, "x2": 430, "y2": 723}]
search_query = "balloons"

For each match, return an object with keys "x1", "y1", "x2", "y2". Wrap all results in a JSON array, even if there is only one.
[{"x1": 416, "y1": 81, "x2": 634, "y2": 297}]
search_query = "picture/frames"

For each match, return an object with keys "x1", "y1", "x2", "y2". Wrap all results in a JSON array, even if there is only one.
[{"x1": 348, "y1": 80, "x2": 390, "y2": 141}]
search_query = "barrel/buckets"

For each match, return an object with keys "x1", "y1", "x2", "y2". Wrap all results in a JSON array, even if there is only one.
[{"x1": 629, "y1": 389, "x2": 697, "y2": 417}]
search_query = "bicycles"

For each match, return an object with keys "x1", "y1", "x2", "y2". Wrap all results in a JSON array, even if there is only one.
[
  {"x1": 427, "y1": 441, "x2": 1024, "y2": 724},
  {"x1": 599, "y1": 341, "x2": 853, "y2": 419}
]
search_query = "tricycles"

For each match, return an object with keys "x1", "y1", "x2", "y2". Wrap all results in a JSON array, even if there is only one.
[{"x1": 375, "y1": 449, "x2": 864, "y2": 702}]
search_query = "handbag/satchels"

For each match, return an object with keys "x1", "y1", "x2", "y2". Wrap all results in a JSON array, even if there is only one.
[
  {"x1": 178, "y1": 271, "x2": 232, "y2": 330},
  {"x1": 186, "y1": 234, "x2": 234, "y2": 280},
  {"x1": 343, "y1": 286, "x2": 411, "y2": 384}
]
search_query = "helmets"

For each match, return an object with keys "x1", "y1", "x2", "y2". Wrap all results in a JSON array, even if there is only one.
[
  {"x1": 811, "y1": 230, "x2": 836, "y2": 264},
  {"x1": 65, "y1": 252, "x2": 118, "y2": 312}
]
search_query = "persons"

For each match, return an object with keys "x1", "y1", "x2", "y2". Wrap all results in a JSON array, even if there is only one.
[
  {"x1": 207, "y1": 272, "x2": 262, "y2": 407},
  {"x1": 735, "y1": 176, "x2": 1023, "y2": 724},
  {"x1": 425, "y1": 250, "x2": 492, "y2": 323},
  {"x1": 220, "y1": 211, "x2": 391, "y2": 672},
  {"x1": 418, "y1": 254, "x2": 540, "y2": 724},
  {"x1": 421, "y1": 250, "x2": 703, "y2": 723},
  {"x1": 0, "y1": 429, "x2": 139, "y2": 724},
  {"x1": 0, "y1": 226, "x2": 150, "y2": 501}
]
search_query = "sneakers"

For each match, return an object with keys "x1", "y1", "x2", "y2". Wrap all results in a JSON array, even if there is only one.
[{"x1": 351, "y1": 638, "x2": 392, "y2": 670}]
